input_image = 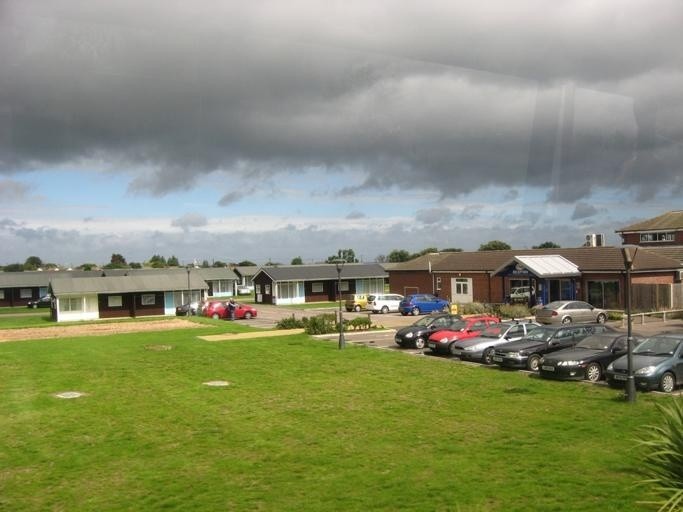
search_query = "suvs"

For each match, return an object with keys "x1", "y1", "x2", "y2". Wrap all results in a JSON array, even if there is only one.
[{"x1": 510, "y1": 285, "x2": 534, "y2": 304}]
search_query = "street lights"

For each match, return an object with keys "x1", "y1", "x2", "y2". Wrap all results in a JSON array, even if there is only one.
[
  {"x1": 620, "y1": 246, "x2": 640, "y2": 402},
  {"x1": 185, "y1": 265, "x2": 191, "y2": 316},
  {"x1": 332, "y1": 258, "x2": 348, "y2": 349}
]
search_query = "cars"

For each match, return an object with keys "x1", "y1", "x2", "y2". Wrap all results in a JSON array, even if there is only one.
[
  {"x1": 174, "y1": 301, "x2": 256, "y2": 320},
  {"x1": 345, "y1": 292, "x2": 451, "y2": 315},
  {"x1": 562, "y1": 288, "x2": 569, "y2": 299},
  {"x1": 393, "y1": 314, "x2": 682, "y2": 392},
  {"x1": 26, "y1": 293, "x2": 50, "y2": 309},
  {"x1": 589, "y1": 289, "x2": 602, "y2": 302},
  {"x1": 536, "y1": 299, "x2": 608, "y2": 326}
]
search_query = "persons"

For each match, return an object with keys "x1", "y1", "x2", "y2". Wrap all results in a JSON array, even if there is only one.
[{"x1": 227, "y1": 296, "x2": 237, "y2": 320}]
